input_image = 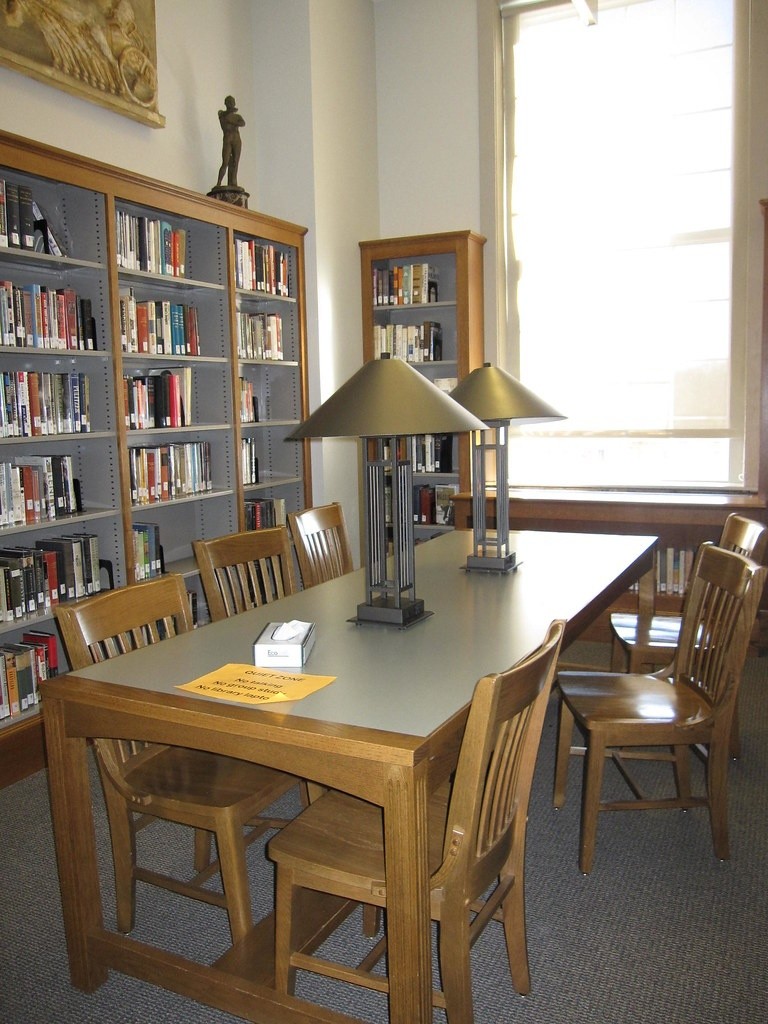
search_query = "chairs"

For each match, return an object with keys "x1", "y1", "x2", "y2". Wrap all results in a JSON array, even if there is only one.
[
  {"x1": 287, "y1": 500, "x2": 355, "y2": 592},
  {"x1": 191, "y1": 526, "x2": 297, "y2": 622},
  {"x1": 267, "y1": 619, "x2": 566, "y2": 1024},
  {"x1": 56, "y1": 572, "x2": 328, "y2": 952},
  {"x1": 556, "y1": 513, "x2": 768, "y2": 872},
  {"x1": 37, "y1": 525, "x2": 657, "y2": 1024}
]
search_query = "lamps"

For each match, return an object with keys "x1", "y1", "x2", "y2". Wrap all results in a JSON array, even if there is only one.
[
  {"x1": 290, "y1": 352, "x2": 490, "y2": 629},
  {"x1": 446, "y1": 363, "x2": 568, "y2": 572}
]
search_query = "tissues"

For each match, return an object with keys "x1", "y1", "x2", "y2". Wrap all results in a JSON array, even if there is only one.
[{"x1": 252, "y1": 619, "x2": 315, "y2": 666}]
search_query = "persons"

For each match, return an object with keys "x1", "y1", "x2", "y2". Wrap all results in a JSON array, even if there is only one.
[{"x1": 214, "y1": 95, "x2": 245, "y2": 187}]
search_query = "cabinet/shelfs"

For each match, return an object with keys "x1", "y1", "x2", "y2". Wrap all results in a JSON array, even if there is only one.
[
  {"x1": 0, "y1": 126, "x2": 309, "y2": 790},
  {"x1": 359, "y1": 230, "x2": 488, "y2": 554}
]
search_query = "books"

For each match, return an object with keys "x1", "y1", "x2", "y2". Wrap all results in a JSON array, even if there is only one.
[
  {"x1": 0, "y1": 177, "x2": 289, "y2": 724},
  {"x1": 372, "y1": 263, "x2": 459, "y2": 558},
  {"x1": 629, "y1": 546, "x2": 693, "y2": 594}
]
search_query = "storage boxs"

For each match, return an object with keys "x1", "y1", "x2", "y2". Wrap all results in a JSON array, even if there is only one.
[{"x1": 254, "y1": 622, "x2": 317, "y2": 668}]
